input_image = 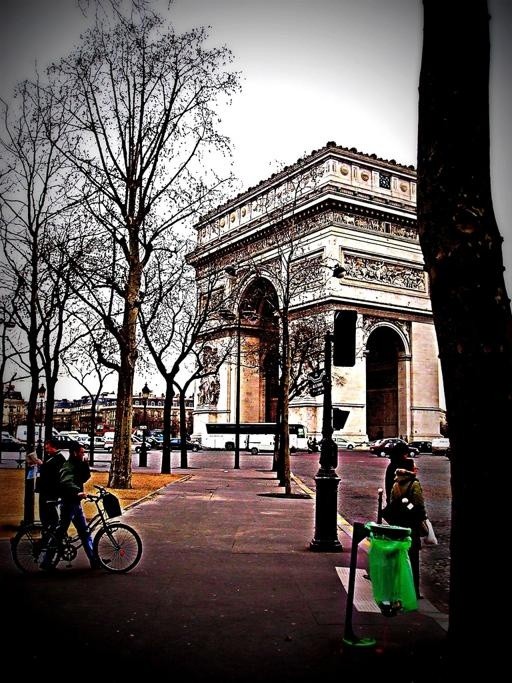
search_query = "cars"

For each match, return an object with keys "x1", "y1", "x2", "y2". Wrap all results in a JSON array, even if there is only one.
[
  {"x1": 369, "y1": 438, "x2": 431, "y2": 458},
  {"x1": 1, "y1": 423, "x2": 105, "y2": 452},
  {"x1": 147, "y1": 433, "x2": 203, "y2": 453},
  {"x1": 333, "y1": 438, "x2": 357, "y2": 449}
]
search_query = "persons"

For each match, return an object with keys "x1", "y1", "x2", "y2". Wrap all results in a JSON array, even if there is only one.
[
  {"x1": 39, "y1": 444, "x2": 112, "y2": 570},
  {"x1": 385, "y1": 443, "x2": 428, "y2": 600},
  {"x1": 28, "y1": 439, "x2": 67, "y2": 526},
  {"x1": 307, "y1": 437, "x2": 319, "y2": 452}
]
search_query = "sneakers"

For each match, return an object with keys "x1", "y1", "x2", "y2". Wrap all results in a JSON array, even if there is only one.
[
  {"x1": 89, "y1": 558, "x2": 111, "y2": 568},
  {"x1": 40, "y1": 561, "x2": 56, "y2": 571}
]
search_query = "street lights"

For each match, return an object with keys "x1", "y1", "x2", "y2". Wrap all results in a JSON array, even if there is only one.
[
  {"x1": 225, "y1": 263, "x2": 347, "y2": 486},
  {"x1": 139, "y1": 382, "x2": 151, "y2": 467},
  {"x1": 37, "y1": 383, "x2": 45, "y2": 459}
]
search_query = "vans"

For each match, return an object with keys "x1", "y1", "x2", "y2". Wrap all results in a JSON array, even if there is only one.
[{"x1": 104, "y1": 432, "x2": 153, "y2": 454}]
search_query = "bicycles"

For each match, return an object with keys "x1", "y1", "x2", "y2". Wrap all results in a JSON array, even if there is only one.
[{"x1": 11, "y1": 485, "x2": 142, "y2": 574}]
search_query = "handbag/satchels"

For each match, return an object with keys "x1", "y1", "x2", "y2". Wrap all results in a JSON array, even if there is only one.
[{"x1": 383, "y1": 493, "x2": 426, "y2": 526}]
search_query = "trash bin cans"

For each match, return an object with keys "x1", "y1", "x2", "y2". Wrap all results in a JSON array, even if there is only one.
[{"x1": 370, "y1": 525, "x2": 418, "y2": 618}]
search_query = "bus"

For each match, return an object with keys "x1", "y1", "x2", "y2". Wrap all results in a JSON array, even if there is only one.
[{"x1": 202, "y1": 423, "x2": 308, "y2": 455}]
